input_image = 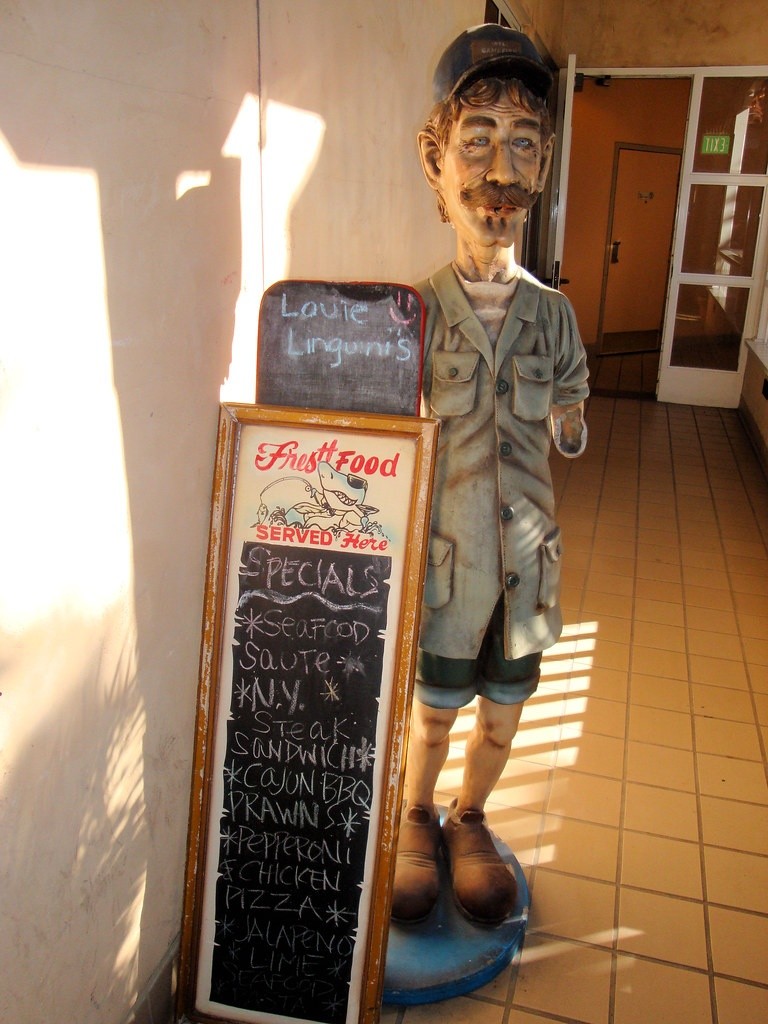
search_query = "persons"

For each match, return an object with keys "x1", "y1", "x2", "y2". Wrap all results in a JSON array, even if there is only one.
[{"x1": 392, "y1": 23, "x2": 593, "y2": 926}]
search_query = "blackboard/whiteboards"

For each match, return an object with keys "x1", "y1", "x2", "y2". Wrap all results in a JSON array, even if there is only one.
[
  {"x1": 175, "y1": 403, "x2": 441, "y2": 1024},
  {"x1": 255, "y1": 279, "x2": 425, "y2": 418}
]
